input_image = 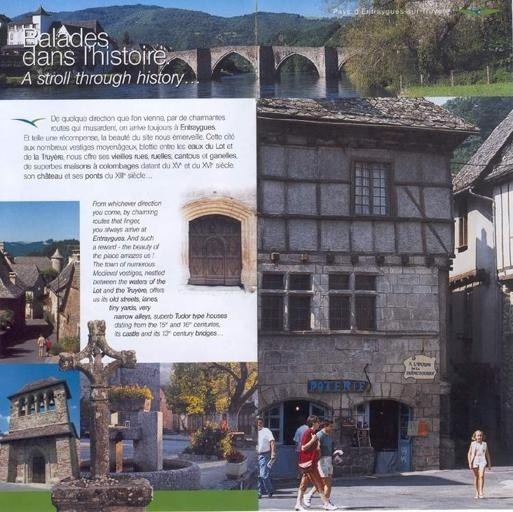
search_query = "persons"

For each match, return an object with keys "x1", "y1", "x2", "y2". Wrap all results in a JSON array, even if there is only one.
[
  {"x1": 294, "y1": 414, "x2": 338, "y2": 511},
  {"x1": 258, "y1": 418, "x2": 276, "y2": 498},
  {"x1": 38, "y1": 333, "x2": 51, "y2": 357},
  {"x1": 468, "y1": 430, "x2": 491, "y2": 498},
  {"x1": 292, "y1": 424, "x2": 309, "y2": 488},
  {"x1": 303, "y1": 420, "x2": 344, "y2": 507}
]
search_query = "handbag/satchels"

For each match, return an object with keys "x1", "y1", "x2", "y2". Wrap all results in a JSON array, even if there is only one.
[{"x1": 297, "y1": 461, "x2": 315, "y2": 474}]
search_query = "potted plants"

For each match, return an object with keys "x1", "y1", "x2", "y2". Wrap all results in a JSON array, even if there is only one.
[
  {"x1": 109, "y1": 384, "x2": 154, "y2": 411},
  {"x1": 226, "y1": 451, "x2": 248, "y2": 479}
]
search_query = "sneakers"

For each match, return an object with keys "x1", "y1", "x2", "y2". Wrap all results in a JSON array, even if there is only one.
[
  {"x1": 303, "y1": 493, "x2": 311, "y2": 507},
  {"x1": 294, "y1": 505, "x2": 307, "y2": 511},
  {"x1": 322, "y1": 503, "x2": 338, "y2": 510}
]
